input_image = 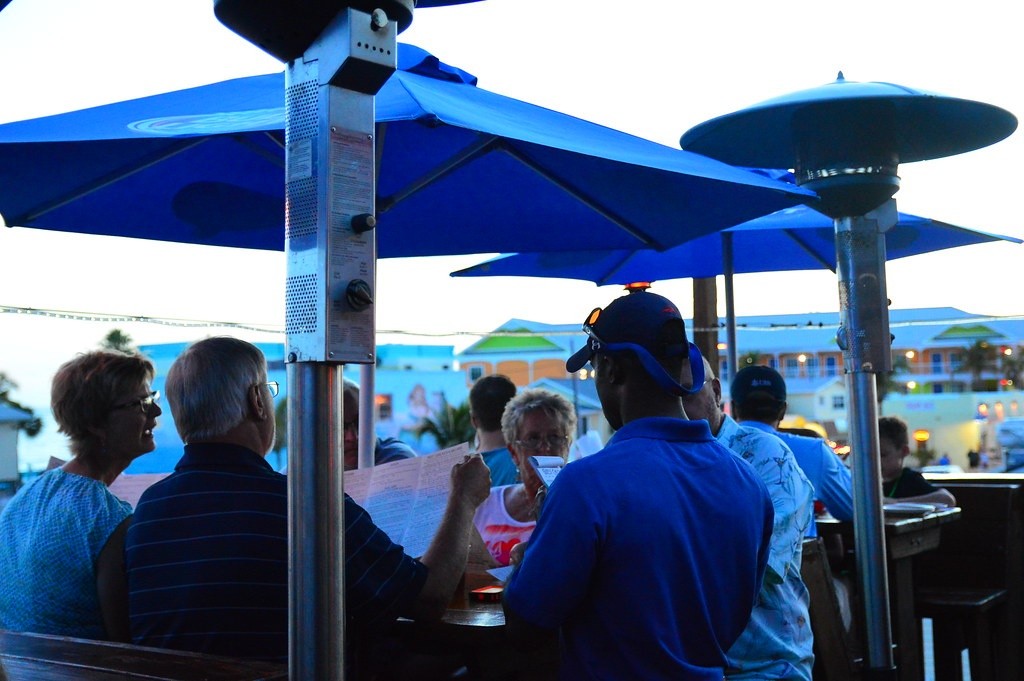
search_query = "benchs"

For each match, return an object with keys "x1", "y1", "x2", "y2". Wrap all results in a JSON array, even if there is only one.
[{"x1": 915, "y1": 483, "x2": 1024, "y2": 681}]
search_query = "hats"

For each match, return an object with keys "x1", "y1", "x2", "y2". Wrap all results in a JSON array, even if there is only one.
[
  {"x1": 566, "y1": 291, "x2": 688, "y2": 373},
  {"x1": 731, "y1": 364, "x2": 786, "y2": 404}
]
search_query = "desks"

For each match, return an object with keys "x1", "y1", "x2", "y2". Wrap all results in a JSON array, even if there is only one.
[
  {"x1": 388, "y1": 560, "x2": 511, "y2": 681},
  {"x1": 814, "y1": 507, "x2": 961, "y2": 681}
]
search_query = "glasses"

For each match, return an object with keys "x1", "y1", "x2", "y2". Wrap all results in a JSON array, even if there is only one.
[
  {"x1": 512, "y1": 432, "x2": 571, "y2": 450},
  {"x1": 105, "y1": 390, "x2": 160, "y2": 417},
  {"x1": 582, "y1": 307, "x2": 606, "y2": 346},
  {"x1": 250, "y1": 382, "x2": 280, "y2": 398}
]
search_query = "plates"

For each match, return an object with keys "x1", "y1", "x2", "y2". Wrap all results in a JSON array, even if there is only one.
[
  {"x1": 910, "y1": 501, "x2": 949, "y2": 509},
  {"x1": 883, "y1": 503, "x2": 935, "y2": 516}
]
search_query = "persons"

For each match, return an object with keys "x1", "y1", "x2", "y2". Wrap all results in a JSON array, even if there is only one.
[
  {"x1": 679, "y1": 355, "x2": 817, "y2": 681},
  {"x1": 939, "y1": 454, "x2": 949, "y2": 465},
  {"x1": 342, "y1": 377, "x2": 418, "y2": 470},
  {"x1": 502, "y1": 293, "x2": 774, "y2": 681},
  {"x1": 968, "y1": 449, "x2": 988, "y2": 471},
  {"x1": 0, "y1": 350, "x2": 162, "y2": 643},
  {"x1": 878, "y1": 416, "x2": 956, "y2": 507},
  {"x1": 471, "y1": 387, "x2": 577, "y2": 567},
  {"x1": 730, "y1": 365, "x2": 853, "y2": 632},
  {"x1": 125, "y1": 338, "x2": 492, "y2": 661},
  {"x1": 468, "y1": 374, "x2": 522, "y2": 486}
]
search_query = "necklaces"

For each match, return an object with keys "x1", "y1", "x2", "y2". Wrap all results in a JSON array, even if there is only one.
[{"x1": 890, "y1": 479, "x2": 899, "y2": 497}]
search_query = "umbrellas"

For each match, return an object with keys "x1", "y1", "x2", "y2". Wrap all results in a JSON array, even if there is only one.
[
  {"x1": 1, "y1": 39, "x2": 820, "y2": 466},
  {"x1": 444, "y1": 165, "x2": 1023, "y2": 392}
]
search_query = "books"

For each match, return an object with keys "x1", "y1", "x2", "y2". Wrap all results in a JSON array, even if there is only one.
[{"x1": 528, "y1": 456, "x2": 564, "y2": 488}]
